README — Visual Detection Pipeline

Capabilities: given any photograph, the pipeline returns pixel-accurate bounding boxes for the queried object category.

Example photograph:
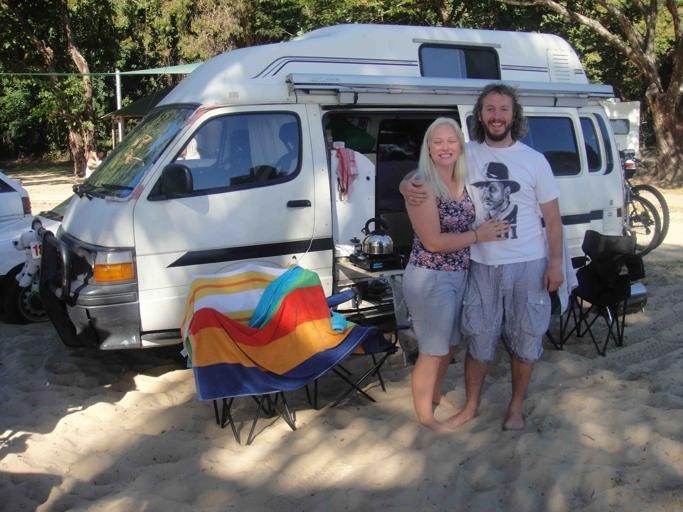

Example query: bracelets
[471,228,478,244]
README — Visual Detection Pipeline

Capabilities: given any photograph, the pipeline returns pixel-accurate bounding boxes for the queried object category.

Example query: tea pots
[361,218,394,255]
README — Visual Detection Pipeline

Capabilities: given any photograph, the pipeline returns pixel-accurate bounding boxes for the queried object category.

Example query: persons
[396,79,567,435]
[84,147,107,179]
[469,162,520,241]
[399,115,510,436]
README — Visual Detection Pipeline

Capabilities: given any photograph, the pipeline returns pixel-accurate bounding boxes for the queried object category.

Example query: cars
[0,192,75,326]
[1,170,35,228]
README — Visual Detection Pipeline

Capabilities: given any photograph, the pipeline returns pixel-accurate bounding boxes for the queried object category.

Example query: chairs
[185,263,394,447]
[274,122,299,174]
[562,230,646,358]
[230,128,251,172]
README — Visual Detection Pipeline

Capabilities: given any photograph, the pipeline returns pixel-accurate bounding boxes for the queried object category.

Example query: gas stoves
[350,249,402,271]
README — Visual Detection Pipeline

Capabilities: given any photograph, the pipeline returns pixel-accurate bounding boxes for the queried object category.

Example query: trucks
[43,28,625,372]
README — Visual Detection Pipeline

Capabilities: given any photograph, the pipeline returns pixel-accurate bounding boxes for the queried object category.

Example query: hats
[471,162,520,194]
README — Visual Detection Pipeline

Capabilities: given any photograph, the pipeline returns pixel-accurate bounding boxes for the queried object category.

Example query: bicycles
[616,146,673,260]
